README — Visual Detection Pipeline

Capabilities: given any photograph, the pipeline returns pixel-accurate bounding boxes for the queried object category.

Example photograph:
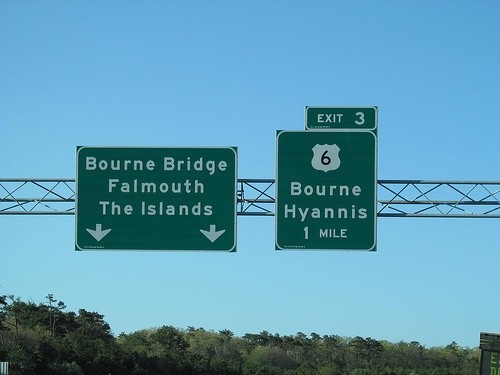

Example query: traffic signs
[275,106,379,251]
[75,144,239,252]
